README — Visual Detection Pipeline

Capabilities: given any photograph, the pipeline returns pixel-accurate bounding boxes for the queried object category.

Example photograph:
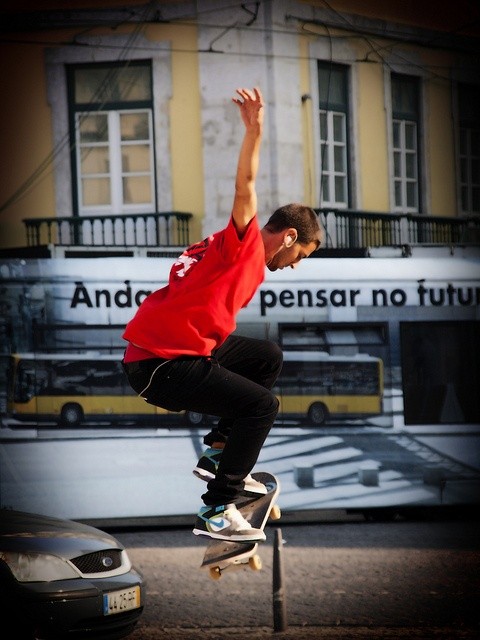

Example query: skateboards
[200,471,281,580]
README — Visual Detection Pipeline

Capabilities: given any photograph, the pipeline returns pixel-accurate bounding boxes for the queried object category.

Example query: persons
[122,84,322,543]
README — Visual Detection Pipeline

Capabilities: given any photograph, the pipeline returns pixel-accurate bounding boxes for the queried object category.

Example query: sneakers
[192,449,268,497]
[193,501,267,543]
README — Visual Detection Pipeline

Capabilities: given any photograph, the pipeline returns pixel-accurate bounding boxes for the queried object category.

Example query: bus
[9,349,383,430]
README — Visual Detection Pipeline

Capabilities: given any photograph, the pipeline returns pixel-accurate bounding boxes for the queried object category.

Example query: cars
[0,505,143,640]
